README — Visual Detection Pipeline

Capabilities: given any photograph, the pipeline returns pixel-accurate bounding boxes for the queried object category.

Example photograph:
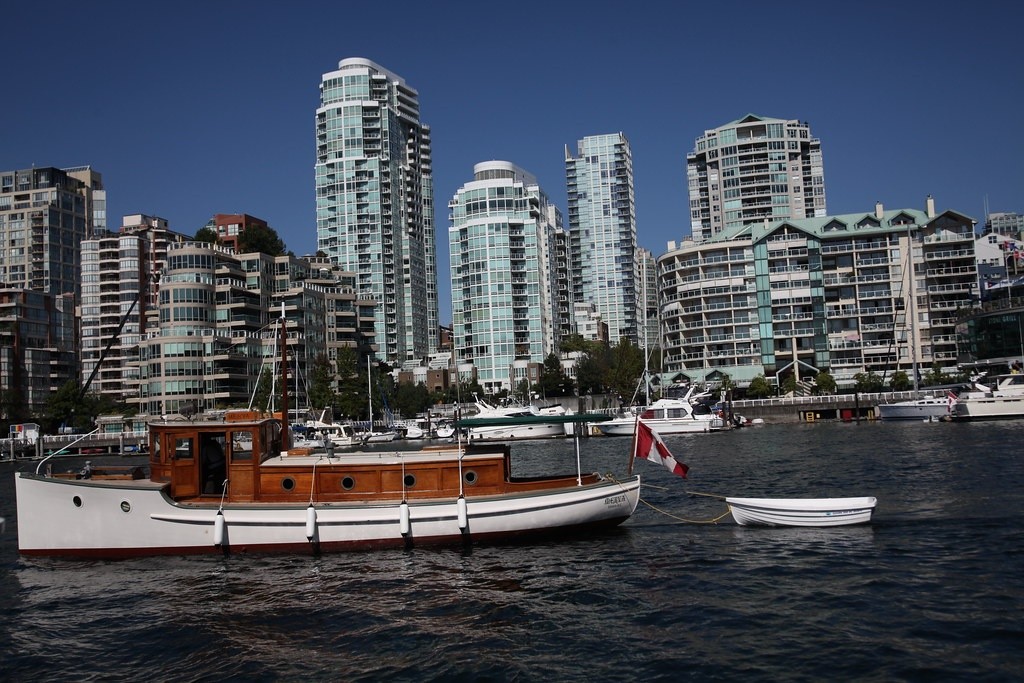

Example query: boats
[15,232,642,562]
[724,496,878,527]
[42,291,754,457]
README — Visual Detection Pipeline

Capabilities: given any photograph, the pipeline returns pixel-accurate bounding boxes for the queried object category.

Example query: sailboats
[873,223,1024,423]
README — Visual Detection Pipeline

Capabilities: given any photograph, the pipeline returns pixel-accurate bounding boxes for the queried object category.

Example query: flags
[1004,240,1015,252]
[1013,246,1024,261]
[635,415,690,482]
[946,390,962,413]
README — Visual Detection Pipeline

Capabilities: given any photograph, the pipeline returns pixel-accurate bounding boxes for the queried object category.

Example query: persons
[81,461,94,479]
[235,443,243,451]
